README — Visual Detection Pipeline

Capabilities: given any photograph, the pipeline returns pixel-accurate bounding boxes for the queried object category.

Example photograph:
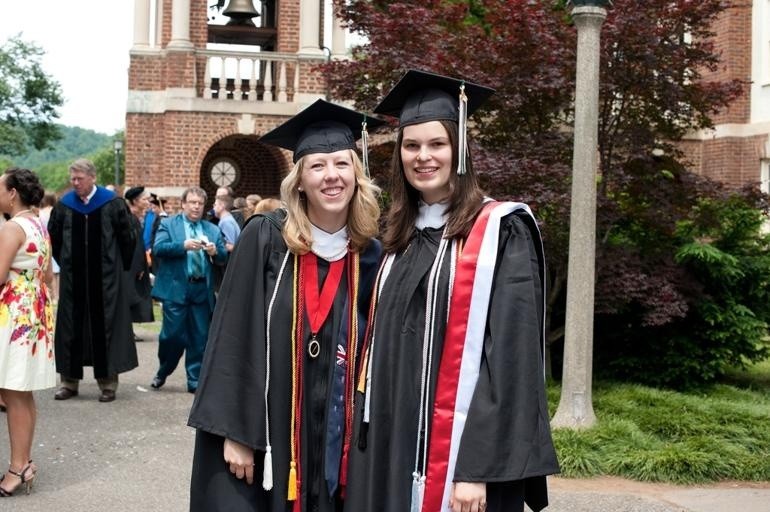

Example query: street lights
[113,139,124,185]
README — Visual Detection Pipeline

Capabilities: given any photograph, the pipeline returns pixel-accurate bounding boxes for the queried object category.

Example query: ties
[190,224,202,280]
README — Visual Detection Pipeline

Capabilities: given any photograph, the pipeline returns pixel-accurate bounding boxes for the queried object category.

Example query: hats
[125,186,144,200]
[256,98,386,182]
[372,69,497,177]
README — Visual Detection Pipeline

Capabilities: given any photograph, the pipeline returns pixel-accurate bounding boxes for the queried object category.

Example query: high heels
[0,459,37,497]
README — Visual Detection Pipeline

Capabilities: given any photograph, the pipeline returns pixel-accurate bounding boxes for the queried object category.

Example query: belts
[189,277,206,283]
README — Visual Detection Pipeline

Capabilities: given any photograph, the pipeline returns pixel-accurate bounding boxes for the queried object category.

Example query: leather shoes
[151,376,165,388]
[55,387,78,400]
[100,389,115,402]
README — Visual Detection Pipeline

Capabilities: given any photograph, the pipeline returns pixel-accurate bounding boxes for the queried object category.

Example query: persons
[1,164,155,411]
[151,187,285,391]
[342,121,560,512]
[1,165,58,499]
[191,148,388,512]
